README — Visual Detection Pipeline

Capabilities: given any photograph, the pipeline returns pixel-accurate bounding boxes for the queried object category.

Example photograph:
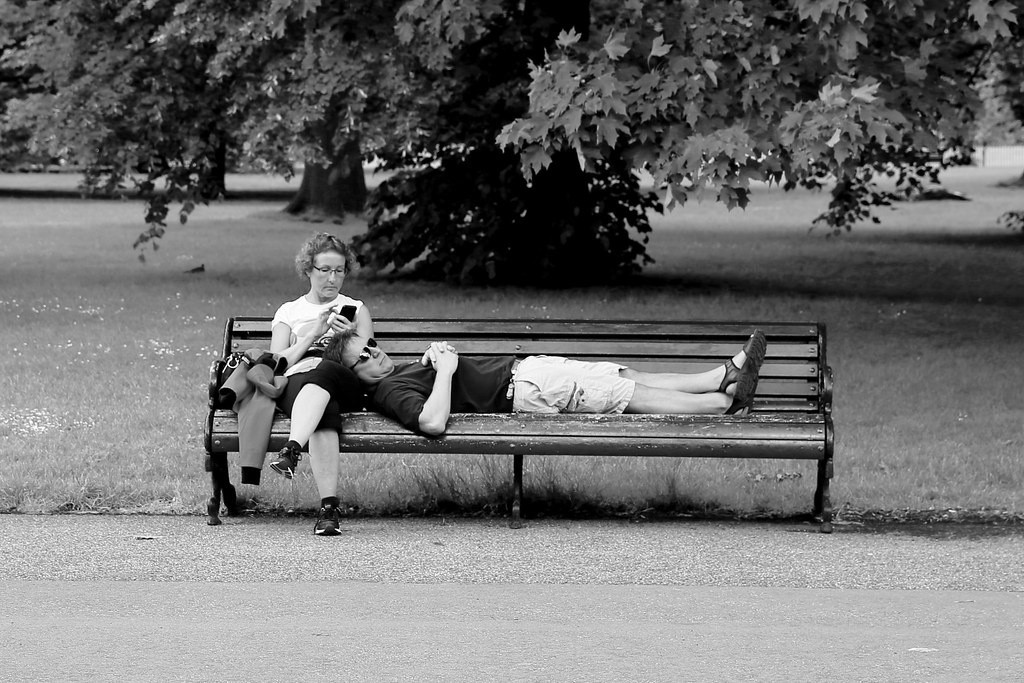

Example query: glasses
[313,266,349,276]
[349,338,377,370]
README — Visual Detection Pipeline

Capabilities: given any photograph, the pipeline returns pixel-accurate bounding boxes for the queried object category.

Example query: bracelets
[425,346,431,351]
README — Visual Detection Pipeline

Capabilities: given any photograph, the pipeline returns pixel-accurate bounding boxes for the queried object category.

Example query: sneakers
[314,505,342,536]
[269,445,301,479]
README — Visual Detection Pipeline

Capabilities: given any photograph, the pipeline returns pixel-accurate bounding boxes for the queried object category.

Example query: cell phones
[336,305,357,324]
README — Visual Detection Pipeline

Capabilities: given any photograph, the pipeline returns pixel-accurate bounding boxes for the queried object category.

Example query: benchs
[203,315,836,535]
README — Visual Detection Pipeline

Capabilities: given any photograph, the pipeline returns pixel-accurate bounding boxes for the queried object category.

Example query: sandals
[719,328,767,415]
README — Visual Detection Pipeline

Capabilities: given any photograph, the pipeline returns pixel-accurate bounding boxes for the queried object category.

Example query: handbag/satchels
[218,352,253,408]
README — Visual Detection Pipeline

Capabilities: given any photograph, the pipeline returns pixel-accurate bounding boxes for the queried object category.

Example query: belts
[510,358,522,380]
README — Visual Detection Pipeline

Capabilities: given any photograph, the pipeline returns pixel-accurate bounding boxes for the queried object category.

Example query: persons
[323,327,768,438]
[269,234,375,535]
